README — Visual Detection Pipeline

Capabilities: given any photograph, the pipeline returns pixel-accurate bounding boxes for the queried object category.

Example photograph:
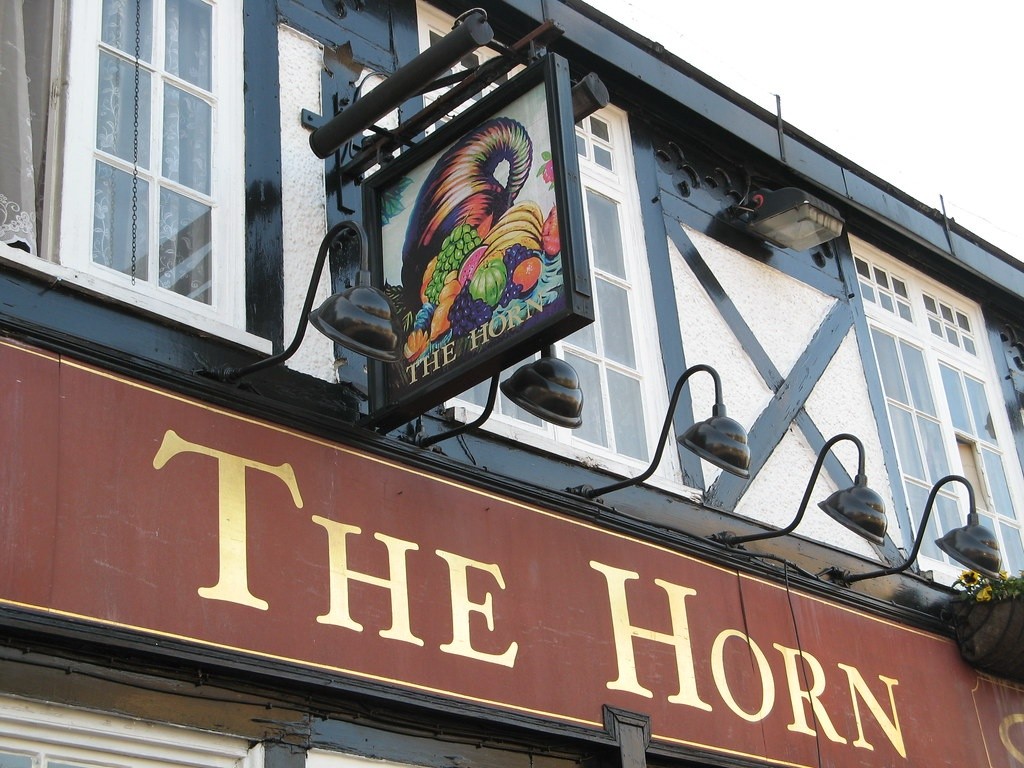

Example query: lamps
[727,184,845,250]
[416,337,582,449]
[710,434,887,552]
[564,364,752,497]
[817,475,1002,581]
[192,222,399,385]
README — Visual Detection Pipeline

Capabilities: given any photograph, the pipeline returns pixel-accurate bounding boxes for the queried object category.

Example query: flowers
[955,569,1024,599]
